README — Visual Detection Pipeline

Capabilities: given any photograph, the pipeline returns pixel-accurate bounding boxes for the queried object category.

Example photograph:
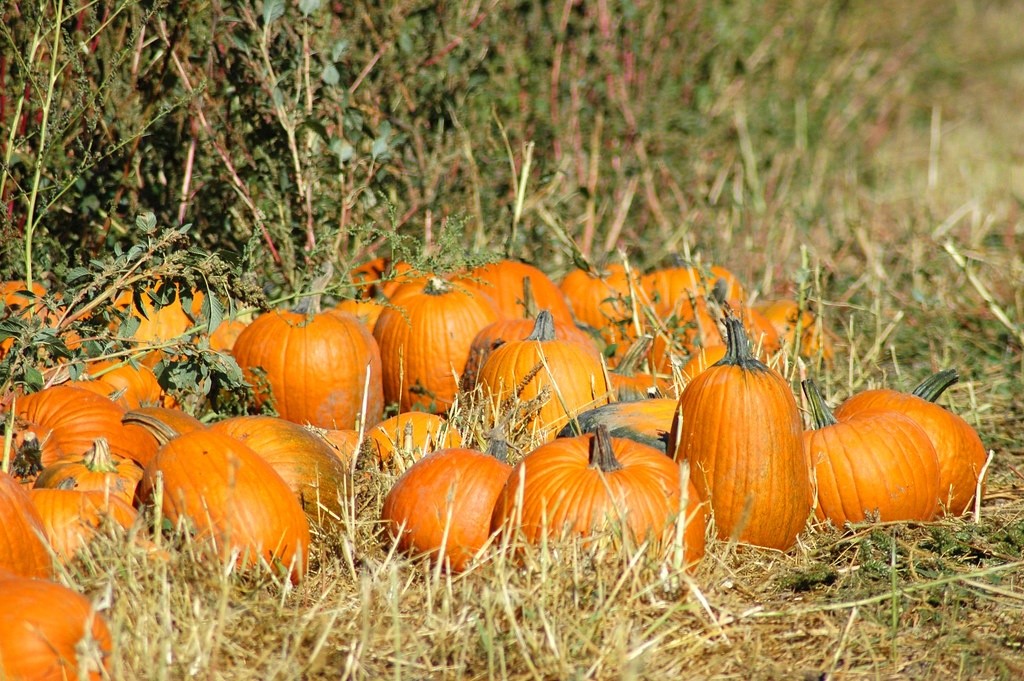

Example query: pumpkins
[0,261,835,681]
[797,378,939,532]
[830,368,988,519]
